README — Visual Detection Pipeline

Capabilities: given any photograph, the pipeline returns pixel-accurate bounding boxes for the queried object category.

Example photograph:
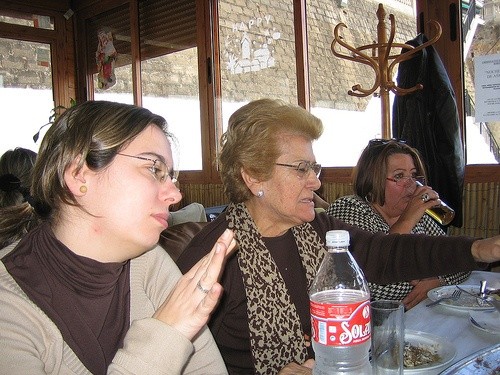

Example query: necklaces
[271,236,290,270]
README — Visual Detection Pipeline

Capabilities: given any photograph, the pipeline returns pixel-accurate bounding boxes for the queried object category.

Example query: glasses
[368,138,407,150]
[275,162,323,180]
[114,151,183,194]
[387,176,426,187]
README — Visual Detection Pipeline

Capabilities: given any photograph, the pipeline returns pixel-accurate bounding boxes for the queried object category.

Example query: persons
[0,101,236,375]
[326,138,471,310]
[175,99,500,375]
[0,147,39,249]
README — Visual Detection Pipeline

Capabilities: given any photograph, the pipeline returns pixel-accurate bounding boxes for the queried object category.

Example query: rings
[421,194,429,202]
[197,280,210,294]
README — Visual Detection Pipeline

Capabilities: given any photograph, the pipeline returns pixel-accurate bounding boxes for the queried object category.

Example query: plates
[469,309,500,335]
[436,343,500,375]
[427,285,500,311]
[376,328,457,372]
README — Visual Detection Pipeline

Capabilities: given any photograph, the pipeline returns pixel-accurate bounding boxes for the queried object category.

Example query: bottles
[307,229,374,375]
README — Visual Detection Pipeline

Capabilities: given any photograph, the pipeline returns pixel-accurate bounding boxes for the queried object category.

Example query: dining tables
[404,270,500,375]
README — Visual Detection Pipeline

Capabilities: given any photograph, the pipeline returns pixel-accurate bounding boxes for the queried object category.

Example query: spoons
[456,285,498,307]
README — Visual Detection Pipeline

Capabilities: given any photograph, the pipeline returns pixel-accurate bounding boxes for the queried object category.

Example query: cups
[407,181,455,226]
[371,300,405,375]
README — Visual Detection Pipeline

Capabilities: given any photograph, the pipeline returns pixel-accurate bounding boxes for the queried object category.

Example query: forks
[425,289,462,307]
